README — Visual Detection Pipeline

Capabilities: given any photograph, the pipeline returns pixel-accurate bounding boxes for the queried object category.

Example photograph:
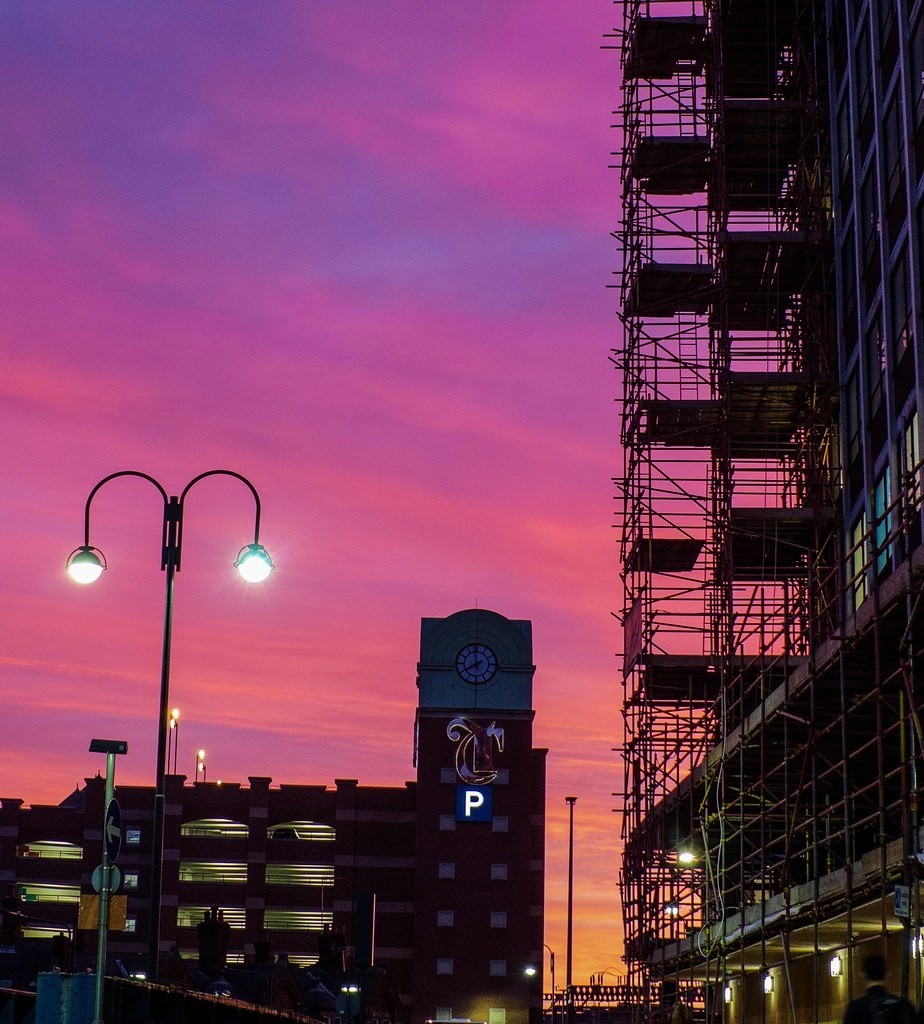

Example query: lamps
[723,987,734,1006]
[763,975,775,994]
[678,851,697,867]
[912,935,924,959]
[829,957,843,977]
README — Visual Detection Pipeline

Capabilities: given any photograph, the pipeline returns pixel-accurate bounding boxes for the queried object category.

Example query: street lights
[63,469,276,981]
[525,943,555,1024]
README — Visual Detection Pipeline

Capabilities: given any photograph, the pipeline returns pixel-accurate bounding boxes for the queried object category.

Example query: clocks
[452,640,500,685]
